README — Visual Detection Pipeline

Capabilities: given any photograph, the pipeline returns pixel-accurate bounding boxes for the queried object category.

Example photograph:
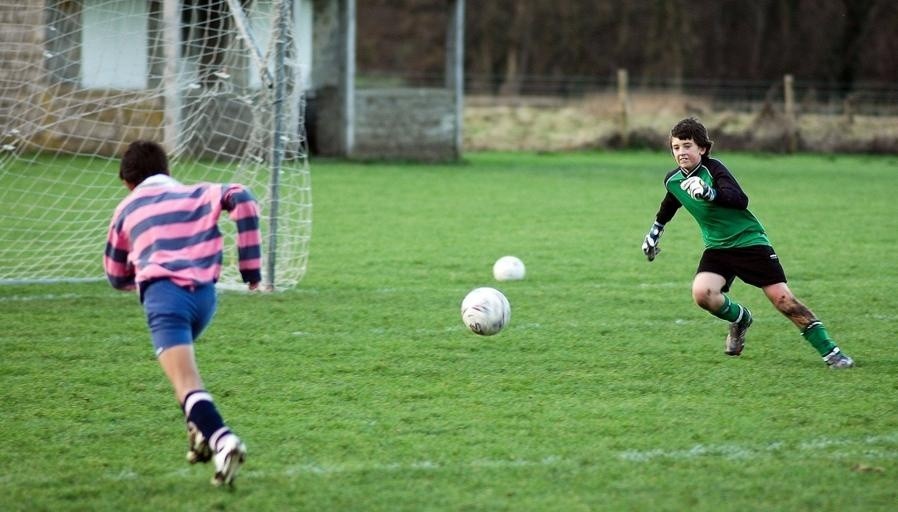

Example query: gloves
[680,176,715,202]
[641,221,665,263]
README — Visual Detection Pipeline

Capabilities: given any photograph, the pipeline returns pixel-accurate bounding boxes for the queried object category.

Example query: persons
[102,139,261,486]
[641,117,854,369]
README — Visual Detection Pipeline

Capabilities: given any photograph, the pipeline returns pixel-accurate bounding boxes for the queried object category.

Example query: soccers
[461,287,511,336]
[492,256,525,281]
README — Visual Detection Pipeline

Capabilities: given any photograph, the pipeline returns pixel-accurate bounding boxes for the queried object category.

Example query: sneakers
[184,421,213,464]
[210,433,248,486]
[826,355,855,368]
[725,304,754,356]
